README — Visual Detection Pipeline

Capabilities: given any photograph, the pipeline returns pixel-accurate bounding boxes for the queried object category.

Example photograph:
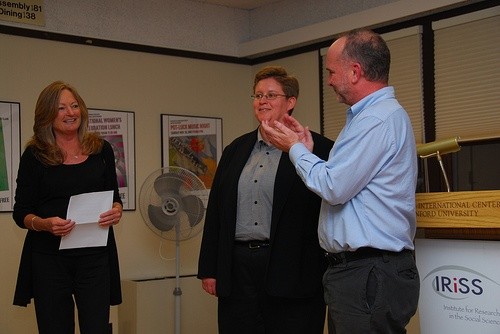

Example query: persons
[13,80,123,334]
[197,65,335,334]
[260,30,420,334]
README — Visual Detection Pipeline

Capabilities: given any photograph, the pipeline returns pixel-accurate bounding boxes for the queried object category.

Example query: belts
[323,248,412,266]
[233,240,272,248]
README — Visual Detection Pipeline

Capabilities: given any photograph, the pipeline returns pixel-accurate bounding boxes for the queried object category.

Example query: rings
[62,233,64,236]
[112,220,115,223]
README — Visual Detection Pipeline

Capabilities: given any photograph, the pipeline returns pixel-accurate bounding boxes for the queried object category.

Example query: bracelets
[32,216,40,232]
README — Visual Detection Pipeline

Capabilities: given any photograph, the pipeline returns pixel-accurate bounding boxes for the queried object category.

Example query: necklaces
[59,143,81,159]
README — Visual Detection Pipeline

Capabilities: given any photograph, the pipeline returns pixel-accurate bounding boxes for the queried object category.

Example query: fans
[138,166,209,334]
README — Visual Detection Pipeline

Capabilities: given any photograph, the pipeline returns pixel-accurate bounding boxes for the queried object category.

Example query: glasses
[251,92,291,100]
